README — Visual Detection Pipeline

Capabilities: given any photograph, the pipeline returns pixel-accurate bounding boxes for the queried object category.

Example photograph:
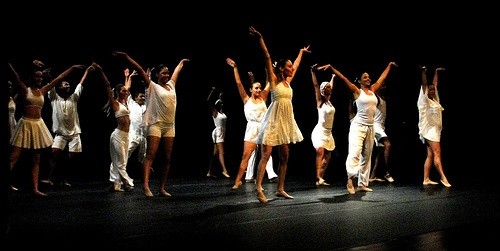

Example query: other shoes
[318,178,326,185]
[11,186,18,190]
[142,191,154,197]
[222,172,231,178]
[347,184,355,195]
[423,180,438,185]
[369,176,385,182]
[35,191,48,196]
[42,180,53,185]
[316,181,331,185]
[440,179,451,188]
[384,173,394,182]
[114,187,124,192]
[357,187,374,192]
[160,190,171,197]
[257,194,267,202]
[276,191,294,199]
[206,173,211,177]
[232,181,242,189]
[126,186,134,192]
[245,178,256,182]
[64,181,71,186]
[269,177,279,182]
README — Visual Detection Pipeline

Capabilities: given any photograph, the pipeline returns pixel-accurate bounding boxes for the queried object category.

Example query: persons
[311,63,336,186]
[418,66,452,189]
[316,62,398,195]
[206,87,232,178]
[111,51,190,198]
[225,57,280,192]
[249,25,311,203]
[3,59,154,197]
